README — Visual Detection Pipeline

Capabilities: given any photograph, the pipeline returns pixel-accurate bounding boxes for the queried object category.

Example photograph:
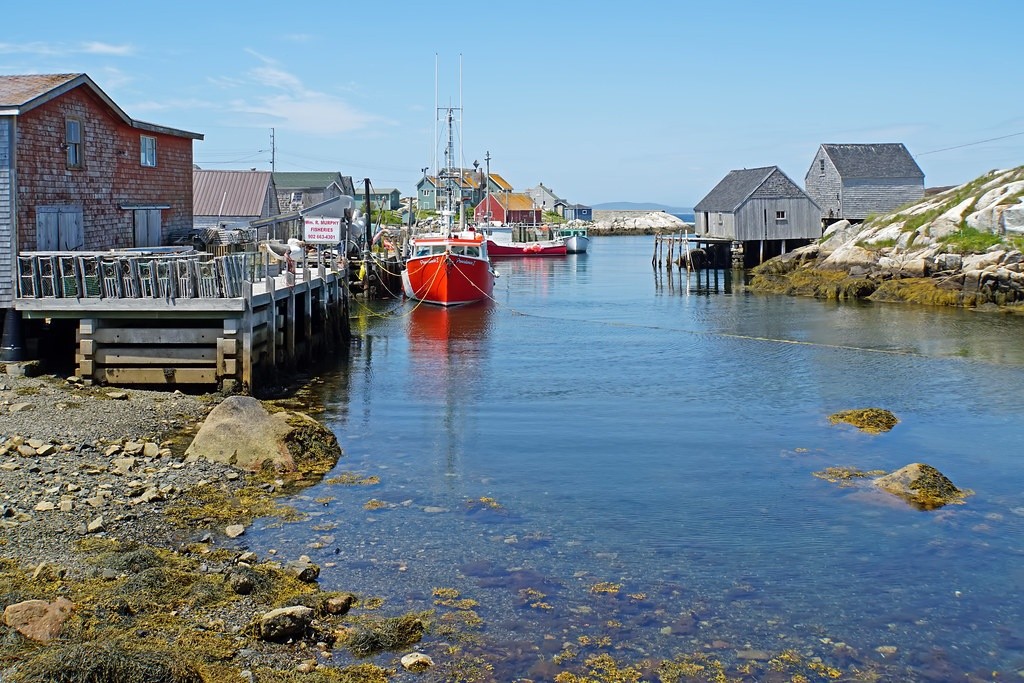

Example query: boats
[401,50,500,308]
[471,151,568,257]
[556,200,589,254]
